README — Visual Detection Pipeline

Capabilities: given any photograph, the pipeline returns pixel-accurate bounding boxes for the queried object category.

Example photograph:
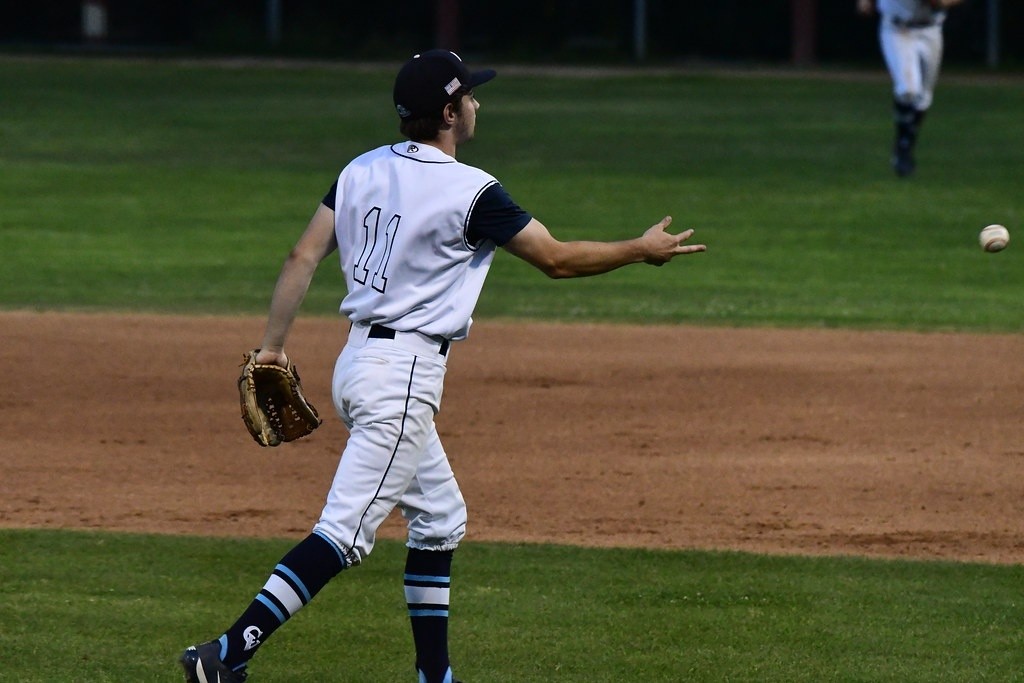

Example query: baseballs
[980,224,1010,252]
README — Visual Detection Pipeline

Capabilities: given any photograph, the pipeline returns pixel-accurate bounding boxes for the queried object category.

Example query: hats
[393,49,497,121]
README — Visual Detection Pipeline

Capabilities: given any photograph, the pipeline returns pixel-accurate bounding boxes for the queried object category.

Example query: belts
[892,16,935,29]
[349,321,449,356]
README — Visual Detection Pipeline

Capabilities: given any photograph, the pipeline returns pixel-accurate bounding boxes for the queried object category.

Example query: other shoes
[182,639,249,683]
[894,139,917,179]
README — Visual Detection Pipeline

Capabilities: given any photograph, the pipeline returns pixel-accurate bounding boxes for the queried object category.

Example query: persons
[858,0,960,179]
[181,50,706,683]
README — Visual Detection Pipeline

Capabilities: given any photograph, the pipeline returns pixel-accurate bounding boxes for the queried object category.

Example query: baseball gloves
[238,348,323,449]
